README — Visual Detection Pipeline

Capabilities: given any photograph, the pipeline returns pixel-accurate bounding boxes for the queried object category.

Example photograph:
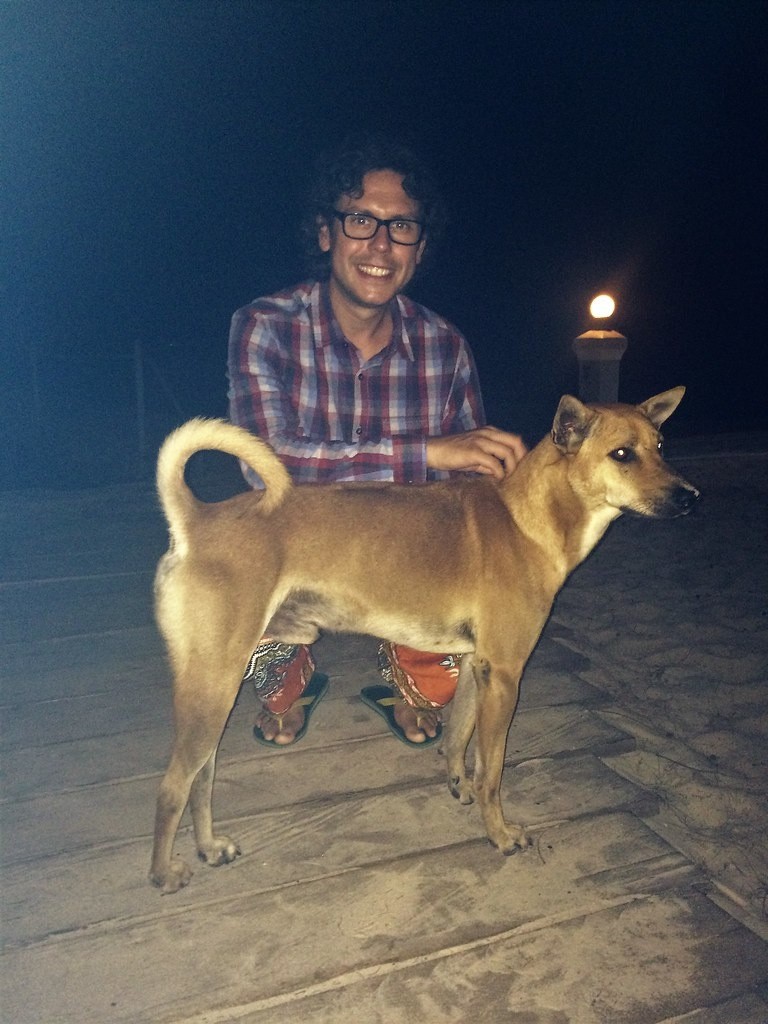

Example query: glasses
[328,205,425,246]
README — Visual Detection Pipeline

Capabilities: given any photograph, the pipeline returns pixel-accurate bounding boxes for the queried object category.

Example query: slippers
[360,684,443,749]
[252,670,330,748]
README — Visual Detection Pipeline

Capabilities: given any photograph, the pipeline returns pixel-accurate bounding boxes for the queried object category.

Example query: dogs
[148,385,700,895]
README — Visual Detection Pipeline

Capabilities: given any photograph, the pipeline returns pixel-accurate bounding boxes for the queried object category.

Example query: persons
[227,153,529,749]
